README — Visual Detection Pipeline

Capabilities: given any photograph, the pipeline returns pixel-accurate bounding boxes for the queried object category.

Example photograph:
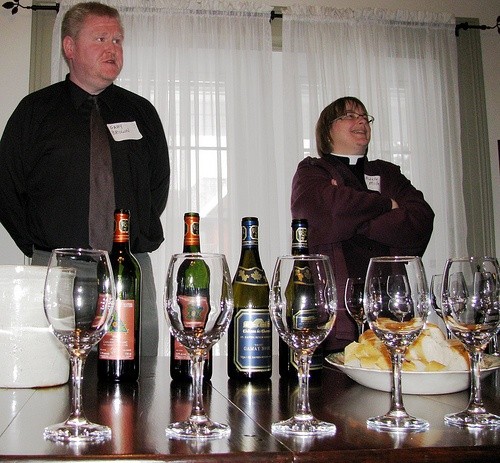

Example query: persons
[0,2,170,358]
[291,97,435,367]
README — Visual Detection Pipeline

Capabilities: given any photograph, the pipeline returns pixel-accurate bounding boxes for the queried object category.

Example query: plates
[326,349,499,395]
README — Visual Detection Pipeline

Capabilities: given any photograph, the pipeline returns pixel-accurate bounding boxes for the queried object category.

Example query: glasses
[331,111,374,123]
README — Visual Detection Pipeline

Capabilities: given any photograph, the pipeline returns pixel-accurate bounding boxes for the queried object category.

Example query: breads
[343,316,471,373]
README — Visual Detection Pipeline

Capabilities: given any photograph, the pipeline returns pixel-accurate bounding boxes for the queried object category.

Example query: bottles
[96,378,136,453]
[170,379,212,454]
[226,377,272,452]
[229,217,272,378]
[279,219,325,374]
[170,212,212,380]
[99,208,141,382]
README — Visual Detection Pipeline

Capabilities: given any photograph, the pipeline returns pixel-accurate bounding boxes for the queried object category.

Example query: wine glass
[44,248,117,447]
[430,272,467,338]
[269,255,338,436]
[164,253,233,442]
[440,258,499,430]
[345,278,382,341]
[364,257,430,432]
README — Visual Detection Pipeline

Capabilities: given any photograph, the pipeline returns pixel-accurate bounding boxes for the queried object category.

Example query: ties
[88,94,116,262]
